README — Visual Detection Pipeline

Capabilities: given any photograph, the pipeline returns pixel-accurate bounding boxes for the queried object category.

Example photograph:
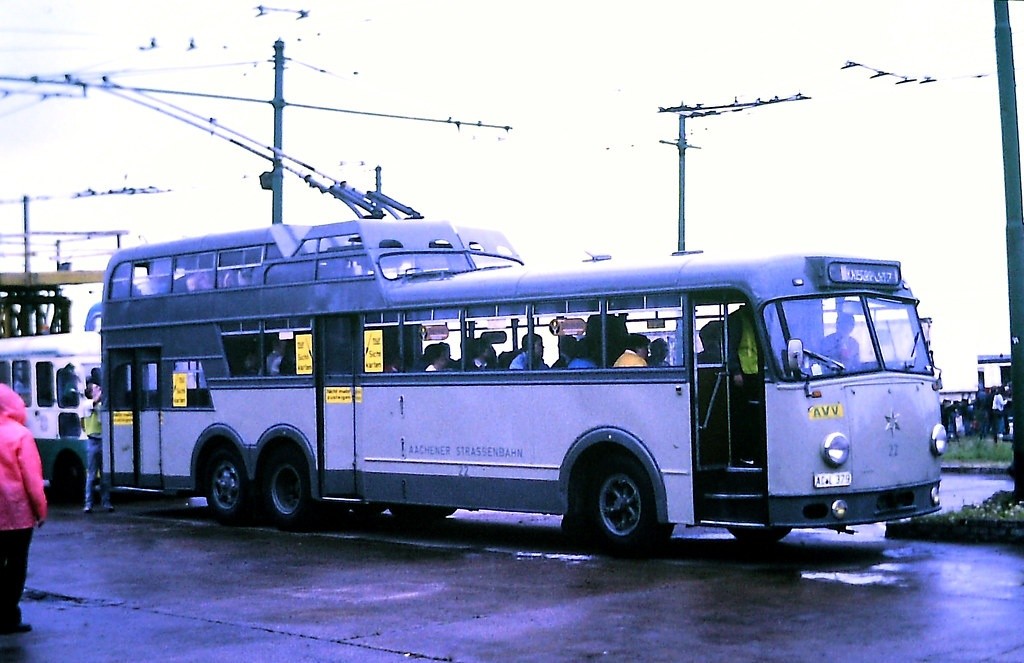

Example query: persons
[612,334,650,369]
[380,338,499,374]
[0,383,49,634]
[257,334,285,376]
[81,383,114,513]
[819,314,860,364]
[551,335,577,368]
[726,300,762,468]
[646,338,671,367]
[568,337,597,367]
[586,313,629,336]
[509,333,550,370]
[940,382,1013,442]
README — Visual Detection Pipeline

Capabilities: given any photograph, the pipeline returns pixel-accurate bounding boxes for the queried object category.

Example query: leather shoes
[0,618,32,638]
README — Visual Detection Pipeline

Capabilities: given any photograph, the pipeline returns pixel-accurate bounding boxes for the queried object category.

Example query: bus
[2,331,103,501]
[96,216,947,553]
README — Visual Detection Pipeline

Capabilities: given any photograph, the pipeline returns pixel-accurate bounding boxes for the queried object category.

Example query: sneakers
[84,505,91,513]
[103,502,113,509]
